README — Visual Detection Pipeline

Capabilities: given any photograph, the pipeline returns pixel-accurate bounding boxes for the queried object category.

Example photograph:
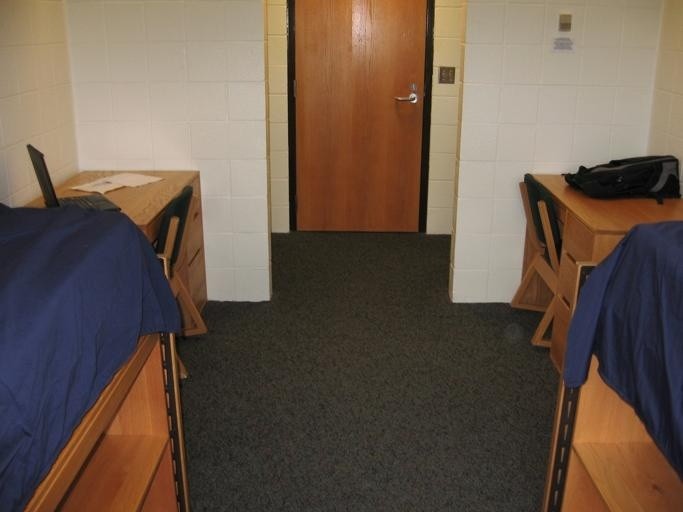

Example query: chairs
[508,172,561,348]
[153,185,209,381]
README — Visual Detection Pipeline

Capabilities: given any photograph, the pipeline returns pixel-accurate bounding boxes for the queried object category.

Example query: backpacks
[564,156,681,205]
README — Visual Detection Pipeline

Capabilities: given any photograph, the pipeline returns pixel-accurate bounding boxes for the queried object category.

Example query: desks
[23,169,208,317]
[518,172,683,376]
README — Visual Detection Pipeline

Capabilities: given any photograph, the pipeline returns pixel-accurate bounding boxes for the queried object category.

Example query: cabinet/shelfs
[22,332,191,512]
[541,259,682,512]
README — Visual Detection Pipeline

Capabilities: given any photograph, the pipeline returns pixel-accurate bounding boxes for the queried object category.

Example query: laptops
[27,144,122,212]
